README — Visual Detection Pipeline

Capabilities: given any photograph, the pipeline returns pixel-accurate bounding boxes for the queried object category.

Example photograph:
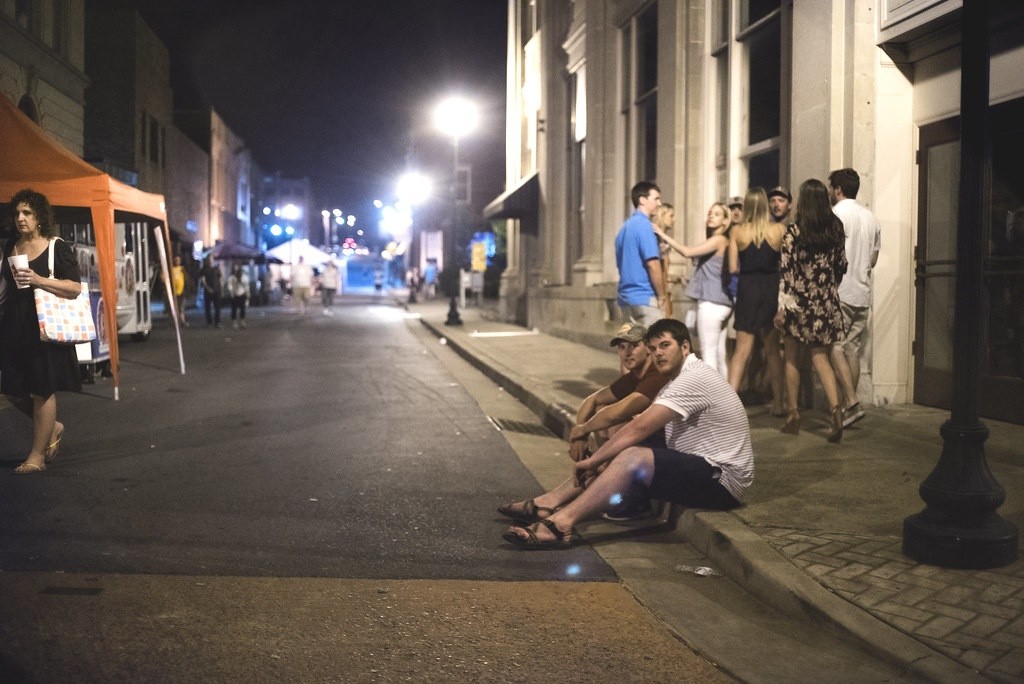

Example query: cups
[7,254,31,288]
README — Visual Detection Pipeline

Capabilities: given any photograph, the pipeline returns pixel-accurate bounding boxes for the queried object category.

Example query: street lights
[401,172,430,303]
[436,97,483,329]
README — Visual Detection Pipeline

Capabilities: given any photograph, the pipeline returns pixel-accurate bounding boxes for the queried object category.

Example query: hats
[769,186,791,202]
[610,323,648,347]
[727,196,743,207]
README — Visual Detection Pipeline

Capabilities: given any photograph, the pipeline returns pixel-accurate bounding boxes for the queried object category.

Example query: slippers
[46,429,64,461]
[12,463,46,476]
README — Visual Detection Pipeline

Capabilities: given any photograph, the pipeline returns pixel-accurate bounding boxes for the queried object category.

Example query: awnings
[482,173,539,220]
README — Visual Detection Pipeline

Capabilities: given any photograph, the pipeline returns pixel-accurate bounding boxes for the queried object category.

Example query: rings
[28,276,29,280]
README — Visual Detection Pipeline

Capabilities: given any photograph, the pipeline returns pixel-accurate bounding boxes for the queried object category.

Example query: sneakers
[603,491,652,520]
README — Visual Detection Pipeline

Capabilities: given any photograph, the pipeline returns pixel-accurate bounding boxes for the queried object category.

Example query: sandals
[503,519,573,549]
[497,499,553,524]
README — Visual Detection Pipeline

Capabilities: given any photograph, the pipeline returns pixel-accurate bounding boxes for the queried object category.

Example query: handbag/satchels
[35,237,97,345]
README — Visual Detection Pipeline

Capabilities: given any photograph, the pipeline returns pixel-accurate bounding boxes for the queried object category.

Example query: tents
[0,88,185,401]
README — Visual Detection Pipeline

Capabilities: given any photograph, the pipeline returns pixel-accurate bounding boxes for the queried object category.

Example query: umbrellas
[202,239,284,264]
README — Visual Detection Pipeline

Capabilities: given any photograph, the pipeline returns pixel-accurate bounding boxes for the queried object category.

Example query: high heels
[827,405,844,441]
[781,408,801,435]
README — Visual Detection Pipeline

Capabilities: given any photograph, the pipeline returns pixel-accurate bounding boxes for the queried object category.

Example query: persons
[0,190,82,474]
[172,256,192,328]
[828,167,880,429]
[613,181,677,374]
[406,260,437,303]
[374,265,383,300]
[227,262,251,328]
[652,202,735,381]
[499,318,754,548]
[773,179,848,443]
[201,253,225,328]
[291,255,314,313]
[321,260,337,319]
[570,323,669,522]
[727,186,794,414]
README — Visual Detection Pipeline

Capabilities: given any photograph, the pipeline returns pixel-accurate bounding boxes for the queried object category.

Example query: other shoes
[233,321,239,329]
[240,318,247,328]
[214,321,222,328]
[323,308,332,316]
[842,402,866,427]
